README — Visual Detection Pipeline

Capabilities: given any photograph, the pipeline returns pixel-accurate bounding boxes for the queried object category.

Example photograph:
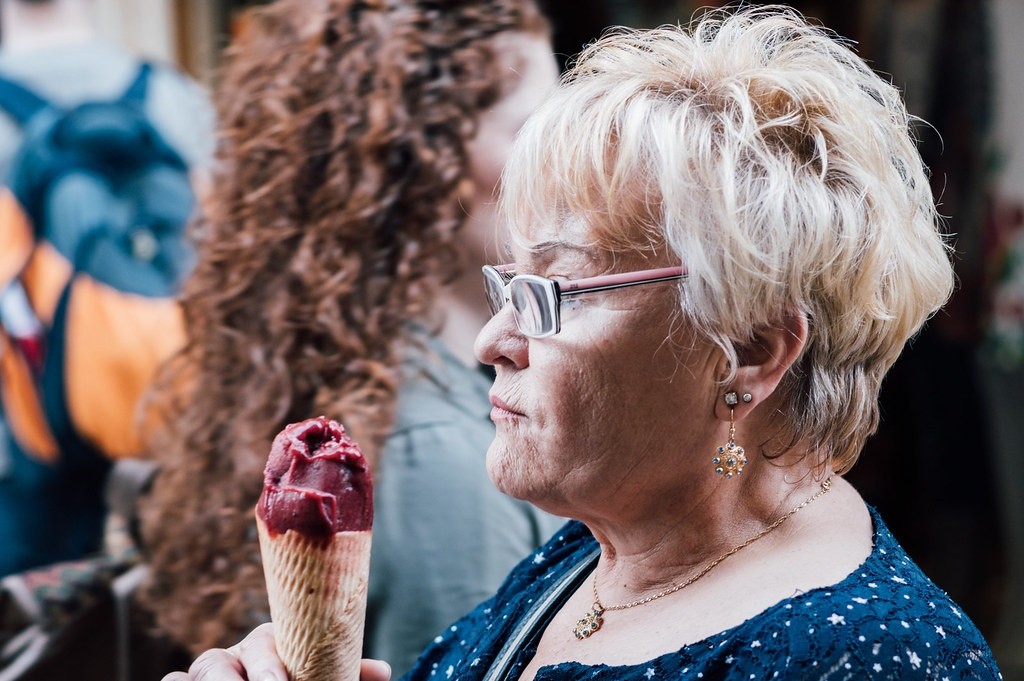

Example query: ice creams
[255,414,373,681]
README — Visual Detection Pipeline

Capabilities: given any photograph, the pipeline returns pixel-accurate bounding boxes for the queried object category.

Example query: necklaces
[572,475,831,641]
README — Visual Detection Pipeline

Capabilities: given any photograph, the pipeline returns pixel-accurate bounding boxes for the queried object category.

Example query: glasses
[482,263,744,338]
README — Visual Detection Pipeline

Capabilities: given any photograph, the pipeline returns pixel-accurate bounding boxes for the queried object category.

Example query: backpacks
[0,59,210,460]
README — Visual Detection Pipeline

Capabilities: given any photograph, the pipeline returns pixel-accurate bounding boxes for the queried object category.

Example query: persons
[160,5,1003,681]
[134,0,563,681]
[0,0,216,576]
